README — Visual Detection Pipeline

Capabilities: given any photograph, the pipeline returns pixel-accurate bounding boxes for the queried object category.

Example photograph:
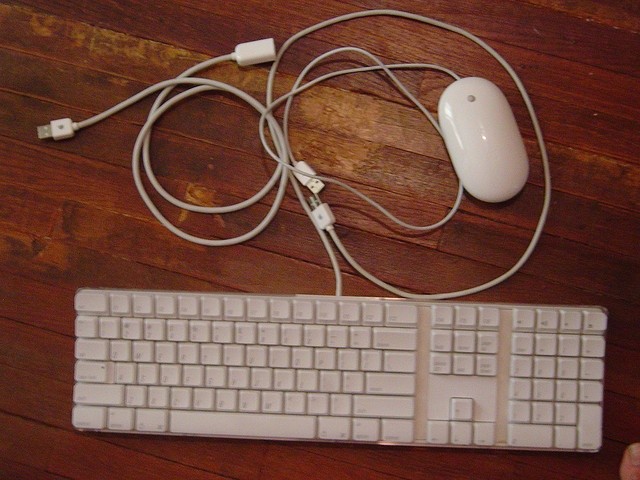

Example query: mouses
[436,76,530,204]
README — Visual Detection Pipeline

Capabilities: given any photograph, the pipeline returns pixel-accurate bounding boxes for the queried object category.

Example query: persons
[619,442,640,480]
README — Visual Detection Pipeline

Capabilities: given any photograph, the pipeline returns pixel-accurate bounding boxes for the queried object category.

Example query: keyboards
[70,283,611,459]
